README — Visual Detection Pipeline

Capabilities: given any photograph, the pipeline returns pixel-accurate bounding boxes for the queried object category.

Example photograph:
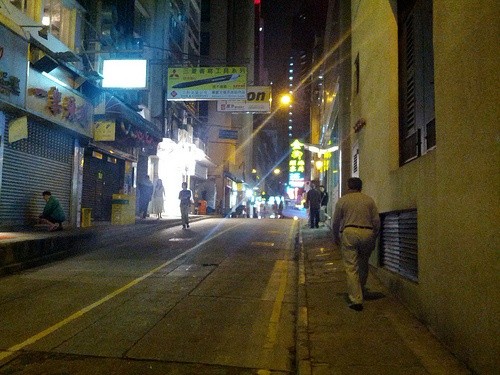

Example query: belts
[344,225,373,230]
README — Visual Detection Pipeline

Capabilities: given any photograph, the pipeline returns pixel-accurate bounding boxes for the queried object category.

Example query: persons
[318,185,331,227]
[153,179,165,219]
[273,201,278,219]
[278,200,284,218]
[332,177,381,311]
[179,182,191,229]
[139,175,153,219]
[38,191,65,230]
[306,183,321,229]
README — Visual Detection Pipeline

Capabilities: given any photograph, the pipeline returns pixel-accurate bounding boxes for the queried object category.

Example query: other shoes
[48,223,59,232]
[187,225,189,227]
[348,303,362,311]
[183,226,186,229]
